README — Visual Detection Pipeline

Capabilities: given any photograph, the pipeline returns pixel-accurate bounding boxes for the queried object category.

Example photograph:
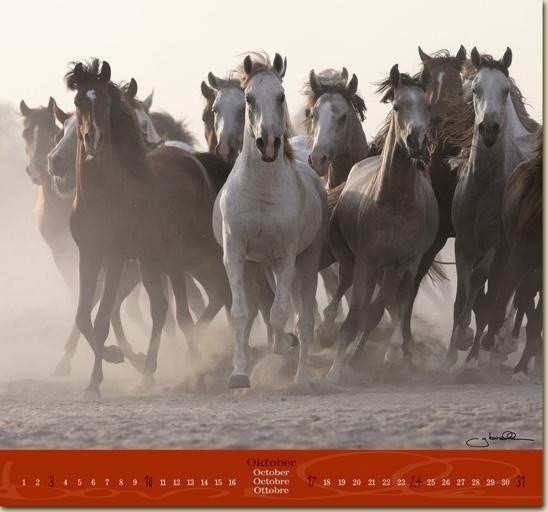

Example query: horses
[11,45,544,406]
